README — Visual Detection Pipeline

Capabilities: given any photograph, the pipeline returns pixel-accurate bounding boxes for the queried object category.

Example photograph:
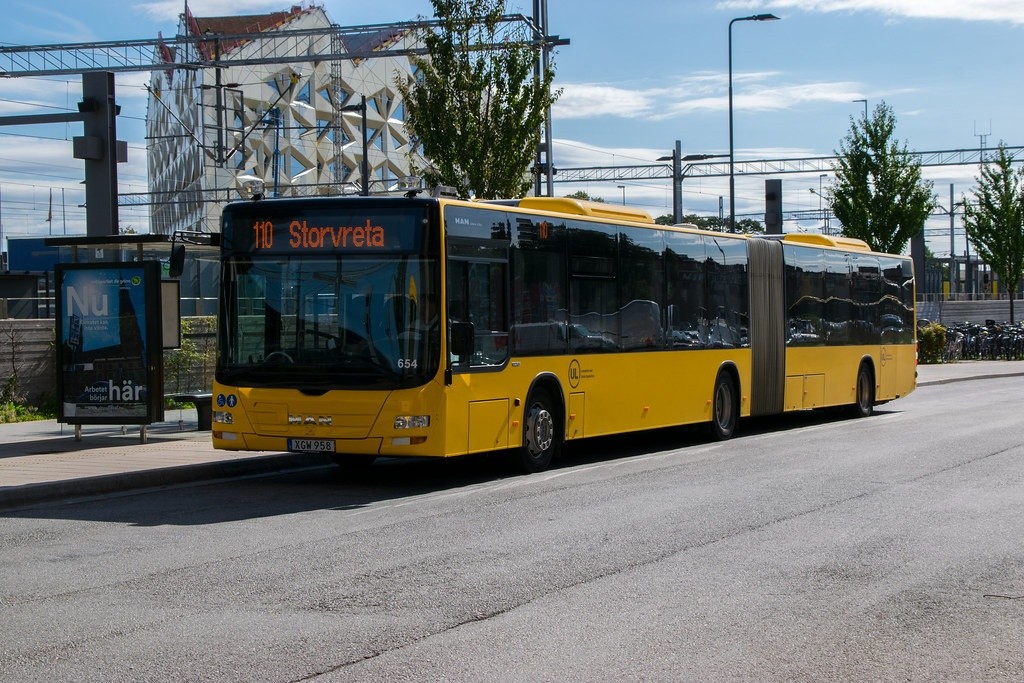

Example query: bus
[168,191,924,474]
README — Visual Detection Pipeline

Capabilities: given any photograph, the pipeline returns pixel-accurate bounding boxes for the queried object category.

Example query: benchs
[173,390,213,430]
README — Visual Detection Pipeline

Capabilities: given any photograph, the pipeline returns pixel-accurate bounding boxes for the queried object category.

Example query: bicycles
[917,317,1024,361]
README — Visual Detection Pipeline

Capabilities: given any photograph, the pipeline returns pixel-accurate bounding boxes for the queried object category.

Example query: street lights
[617,185,627,206]
[925,184,966,299]
[853,98,870,146]
[819,174,829,222]
[336,92,369,198]
[682,13,784,232]
